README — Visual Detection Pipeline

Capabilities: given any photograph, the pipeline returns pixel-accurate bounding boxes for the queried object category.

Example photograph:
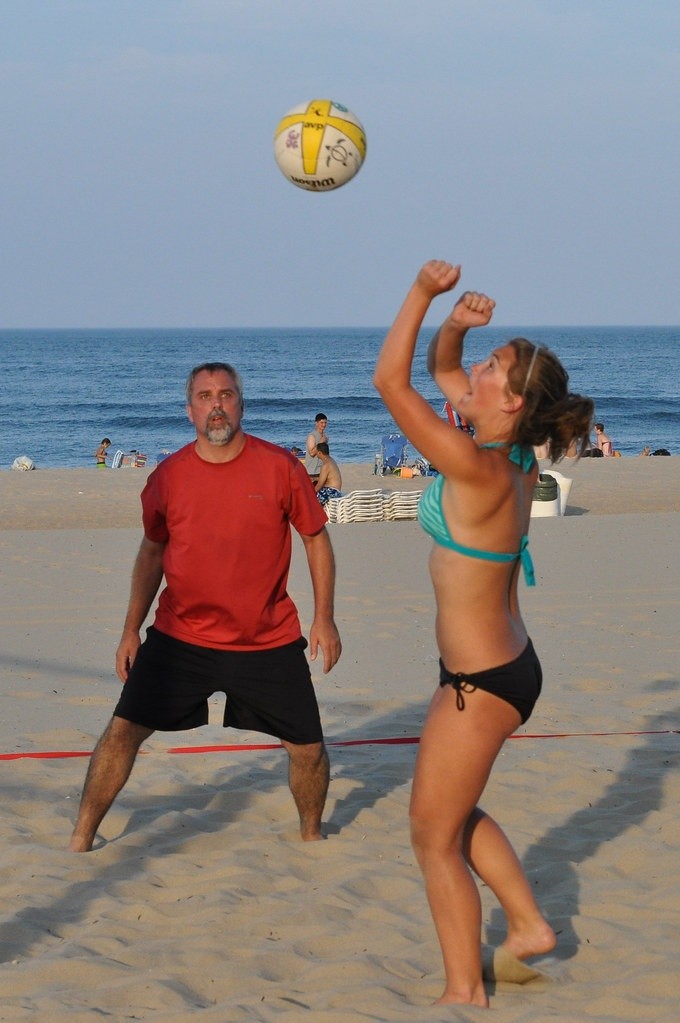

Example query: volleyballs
[272,97,369,194]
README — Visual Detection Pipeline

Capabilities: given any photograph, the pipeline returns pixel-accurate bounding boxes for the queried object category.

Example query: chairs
[376,435,408,478]
[322,489,423,525]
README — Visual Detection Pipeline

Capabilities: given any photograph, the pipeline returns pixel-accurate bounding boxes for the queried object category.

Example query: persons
[591,423,612,457]
[641,445,670,456]
[563,439,577,458]
[287,447,297,456]
[71,362,342,852]
[373,260,593,1005]
[580,441,604,458]
[305,413,329,487]
[96,438,111,468]
[314,443,342,505]
[156,449,171,464]
[534,443,550,459]
